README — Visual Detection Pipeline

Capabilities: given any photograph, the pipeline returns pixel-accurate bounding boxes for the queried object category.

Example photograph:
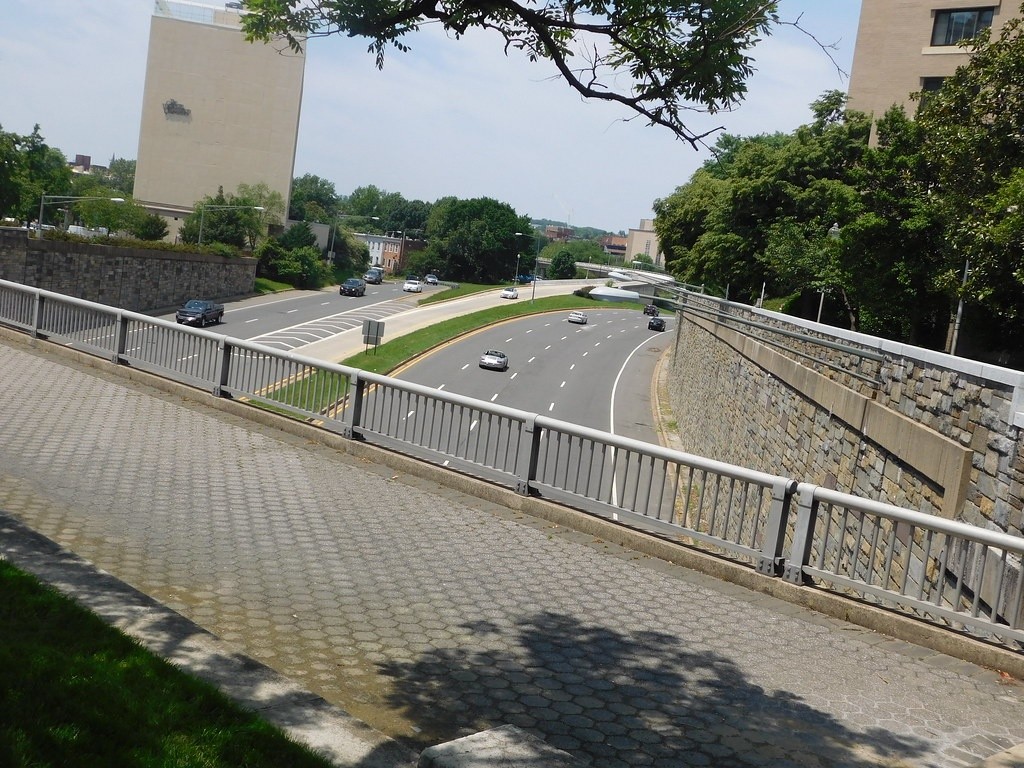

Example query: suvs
[424,275,438,285]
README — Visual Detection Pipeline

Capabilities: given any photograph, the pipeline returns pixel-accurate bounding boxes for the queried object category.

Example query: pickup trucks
[175,300,225,325]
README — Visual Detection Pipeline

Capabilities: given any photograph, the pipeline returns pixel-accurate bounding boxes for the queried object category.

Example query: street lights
[585,256,592,280]
[328,213,380,268]
[36,195,125,238]
[198,202,265,246]
[515,232,542,301]
[514,254,520,287]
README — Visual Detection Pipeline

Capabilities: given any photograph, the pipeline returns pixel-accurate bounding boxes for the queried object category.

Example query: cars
[643,304,661,317]
[478,349,508,373]
[500,288,519,299]
[340,278,366,297]
[403,280,423,293]
[568,311,588,324]
[648,318,666,332]
[363,269,380,284]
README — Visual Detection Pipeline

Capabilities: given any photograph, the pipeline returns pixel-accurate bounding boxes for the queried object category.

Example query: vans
[372,266,385,282]
